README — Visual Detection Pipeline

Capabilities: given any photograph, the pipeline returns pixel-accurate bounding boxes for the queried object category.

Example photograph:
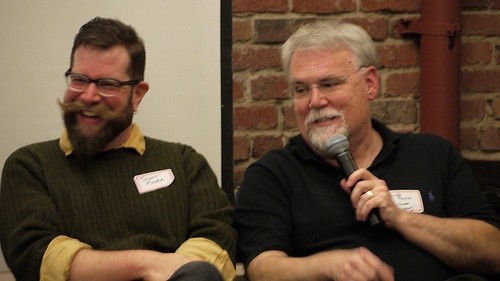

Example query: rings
[365,190,373,199]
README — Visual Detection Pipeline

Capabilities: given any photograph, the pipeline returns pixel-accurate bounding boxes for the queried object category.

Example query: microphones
[323,134,384,228]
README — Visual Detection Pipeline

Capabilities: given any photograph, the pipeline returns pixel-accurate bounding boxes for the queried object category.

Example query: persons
[0,16,236,281]
[234,20,500,281]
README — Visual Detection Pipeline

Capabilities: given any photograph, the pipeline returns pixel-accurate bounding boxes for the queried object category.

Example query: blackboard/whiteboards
[0,0,234,207]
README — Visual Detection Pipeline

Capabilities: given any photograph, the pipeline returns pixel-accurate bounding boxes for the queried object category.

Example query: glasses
[65,68,142,95]
[283,64,369,100]
